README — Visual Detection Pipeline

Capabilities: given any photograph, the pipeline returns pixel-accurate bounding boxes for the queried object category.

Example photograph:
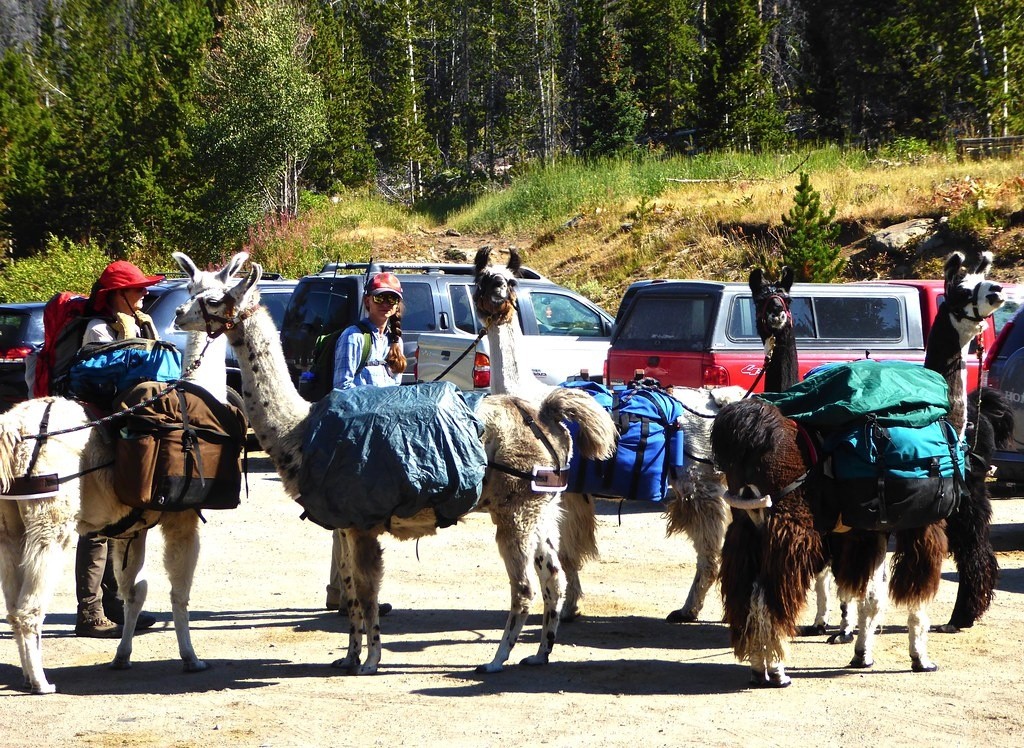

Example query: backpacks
[303,322,372,402]
[25,291,88,404]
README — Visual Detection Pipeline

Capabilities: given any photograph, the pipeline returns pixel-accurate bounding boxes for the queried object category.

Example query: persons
[74,259,157,638]
[325,273,409,616]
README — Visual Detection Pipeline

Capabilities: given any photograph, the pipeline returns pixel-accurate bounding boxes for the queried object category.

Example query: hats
[99,260,166,293]
[366,272,404,300]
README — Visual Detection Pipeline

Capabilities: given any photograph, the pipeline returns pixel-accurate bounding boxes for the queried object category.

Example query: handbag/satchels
[558,381,682,503]
[69,337,182,398]
[112,380,246,510]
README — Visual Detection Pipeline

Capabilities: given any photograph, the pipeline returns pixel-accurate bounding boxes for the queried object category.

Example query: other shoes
[104,605,156,630]
[338,603,391,618]
[76,618,132,638]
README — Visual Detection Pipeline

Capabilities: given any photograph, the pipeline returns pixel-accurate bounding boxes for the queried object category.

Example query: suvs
[281,261,615,403]
[971,305,1024,498]
[140,272,301,431]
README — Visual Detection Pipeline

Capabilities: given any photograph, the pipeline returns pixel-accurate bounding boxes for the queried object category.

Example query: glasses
[125,288,145,293]
[373,294,399,305]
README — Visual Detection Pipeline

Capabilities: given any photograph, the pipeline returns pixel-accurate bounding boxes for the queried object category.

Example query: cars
[0,302,50,414]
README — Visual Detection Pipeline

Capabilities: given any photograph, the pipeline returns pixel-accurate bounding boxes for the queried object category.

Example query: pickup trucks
[414,332,611,400]
[601,277,1023,483]
